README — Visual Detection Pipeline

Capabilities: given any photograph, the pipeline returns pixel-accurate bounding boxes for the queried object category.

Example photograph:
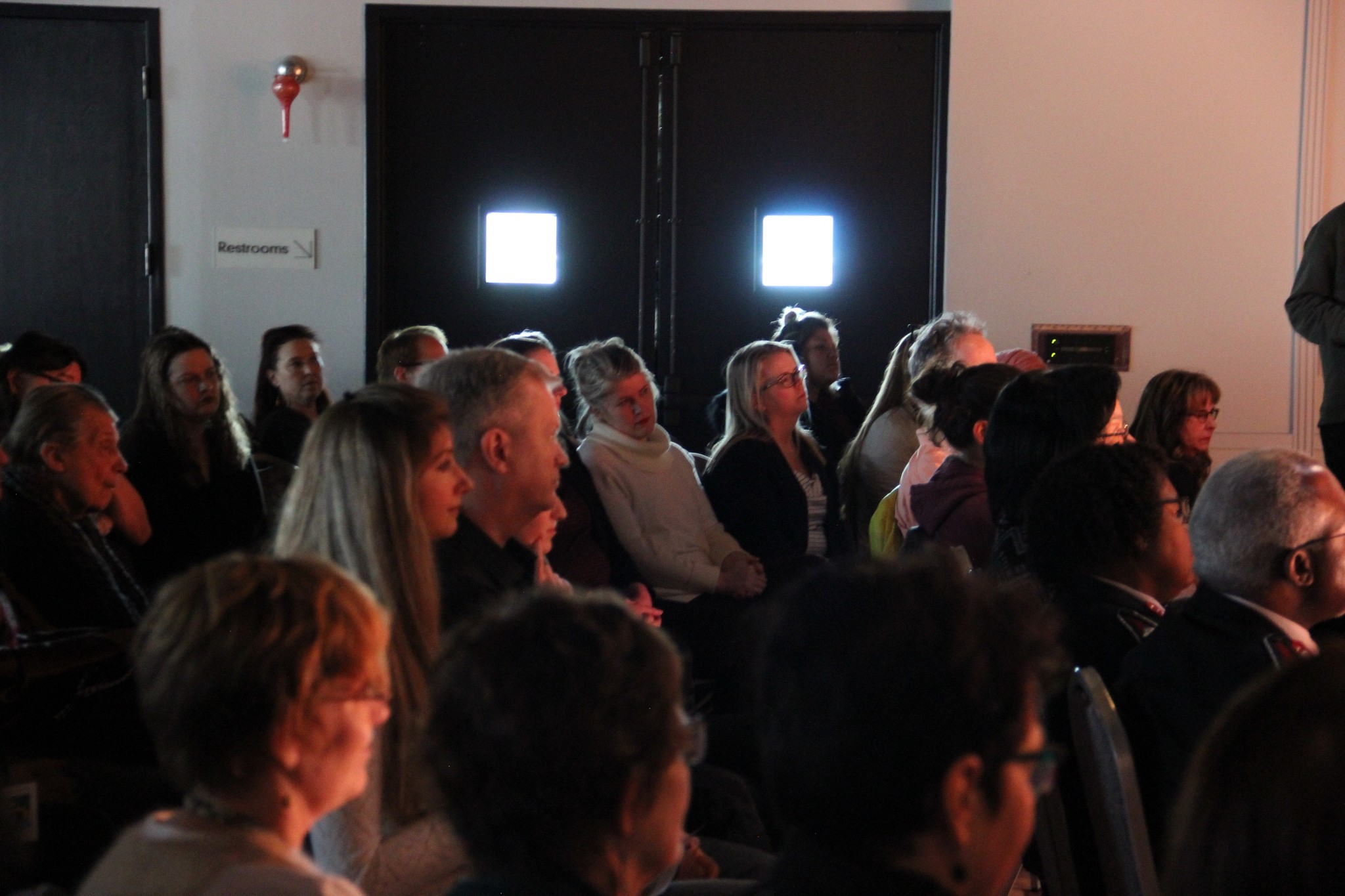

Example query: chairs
[1050,662,1171,896]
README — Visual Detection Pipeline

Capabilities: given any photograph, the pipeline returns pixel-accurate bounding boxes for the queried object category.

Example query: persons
[1285,203,1345,489]
[0,307,1345,896]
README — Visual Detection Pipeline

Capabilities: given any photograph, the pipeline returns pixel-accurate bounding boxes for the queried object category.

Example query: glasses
[1145,494,1190,521]
[758,363,809,395]
[1097,423,1129,440]
[1184,408,1219,424]
[649,719,707,767]
[997,747,1054,788]
[314,682,387,704]
